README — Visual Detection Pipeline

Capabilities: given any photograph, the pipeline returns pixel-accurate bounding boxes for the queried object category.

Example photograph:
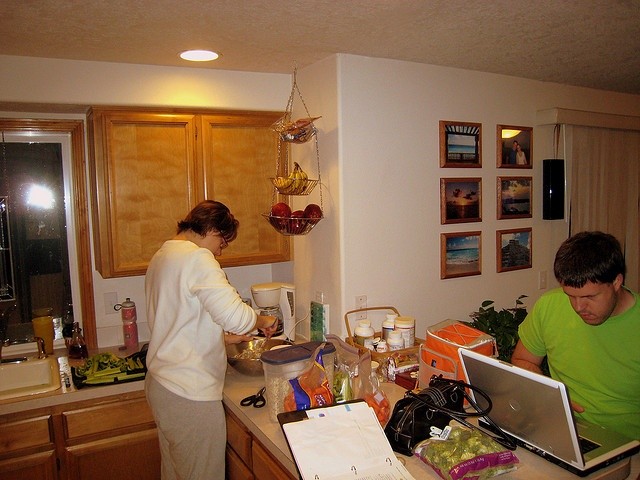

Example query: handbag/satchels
[383,373,517,457]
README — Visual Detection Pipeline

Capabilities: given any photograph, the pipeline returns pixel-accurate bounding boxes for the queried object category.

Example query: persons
[143,200,279,479]
[515,144,528,165]
[506,140,518,165]
[509,230,639,445]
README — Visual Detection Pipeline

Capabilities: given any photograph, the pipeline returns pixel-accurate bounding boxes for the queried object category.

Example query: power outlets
[103,291,119,314]
[354,295,367,320]
[539,270,548,289]
[314,289,323,303]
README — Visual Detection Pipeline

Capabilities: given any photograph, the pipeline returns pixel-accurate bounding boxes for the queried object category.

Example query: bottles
[68,321,90,369]
[394,316,414,349]
[62,303,73,339]
[372,337,381,351]
[387,330,404,352]
[376,338,388,354]
[354,318,375,347]
[57,356,75,393]
[52,317,64,340]
[383,312,399,341]
[121,298,139,350]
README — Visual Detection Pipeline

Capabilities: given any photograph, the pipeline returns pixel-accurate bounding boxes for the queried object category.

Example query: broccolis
[412,426,520,479]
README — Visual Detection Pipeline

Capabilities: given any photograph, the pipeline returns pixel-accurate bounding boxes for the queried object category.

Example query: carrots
[360,391,390,428]
[282,383,334,414]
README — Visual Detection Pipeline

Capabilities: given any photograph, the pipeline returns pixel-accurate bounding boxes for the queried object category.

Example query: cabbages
[330,370,355,404]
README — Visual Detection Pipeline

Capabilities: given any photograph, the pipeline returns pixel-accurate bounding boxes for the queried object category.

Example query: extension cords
[309,300,329,341]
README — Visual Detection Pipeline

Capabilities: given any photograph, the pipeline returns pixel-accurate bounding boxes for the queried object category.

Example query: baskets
[261,212,324,236]
[344,307,426,374]
[268,176,320,196]
[269,116,318,143]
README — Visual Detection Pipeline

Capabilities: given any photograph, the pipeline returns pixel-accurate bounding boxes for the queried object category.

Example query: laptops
[457,347,640,477]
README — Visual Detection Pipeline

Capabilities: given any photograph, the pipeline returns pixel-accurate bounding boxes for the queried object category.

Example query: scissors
[241,386,267,408]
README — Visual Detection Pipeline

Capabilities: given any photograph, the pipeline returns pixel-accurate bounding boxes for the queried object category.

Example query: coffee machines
[249,282,296,343]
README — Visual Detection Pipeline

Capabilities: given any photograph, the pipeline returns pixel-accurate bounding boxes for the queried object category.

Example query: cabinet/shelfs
[0,390,162,480]
[221,396,298,480]
[85,106,293,280]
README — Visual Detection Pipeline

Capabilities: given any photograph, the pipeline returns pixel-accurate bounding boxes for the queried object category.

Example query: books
[276,398,416,480]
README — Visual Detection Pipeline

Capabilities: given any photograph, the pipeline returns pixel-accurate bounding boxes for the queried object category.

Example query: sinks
[0,354,61,401]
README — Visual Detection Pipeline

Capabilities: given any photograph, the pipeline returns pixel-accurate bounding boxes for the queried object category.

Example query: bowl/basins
[224,338,296,377]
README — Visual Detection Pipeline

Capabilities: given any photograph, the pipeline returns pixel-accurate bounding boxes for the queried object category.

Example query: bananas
[271,161,309,195]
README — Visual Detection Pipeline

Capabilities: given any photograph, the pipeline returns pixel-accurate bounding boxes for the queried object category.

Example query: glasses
[219,229,228,249]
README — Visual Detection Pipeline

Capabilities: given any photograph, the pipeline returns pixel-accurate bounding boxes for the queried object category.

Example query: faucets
[32,335,47,360]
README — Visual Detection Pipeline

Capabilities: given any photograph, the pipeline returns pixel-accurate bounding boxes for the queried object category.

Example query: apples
[269,202,292,230]
[287,210,307,235]
[304,203,322,225]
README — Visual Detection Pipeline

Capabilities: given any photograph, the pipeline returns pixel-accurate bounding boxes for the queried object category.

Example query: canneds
[122,321,139,350]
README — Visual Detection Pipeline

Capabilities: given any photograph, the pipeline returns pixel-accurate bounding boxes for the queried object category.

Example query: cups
[32,316,54,356]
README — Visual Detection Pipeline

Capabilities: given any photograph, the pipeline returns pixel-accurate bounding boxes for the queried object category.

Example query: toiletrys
[69,322,88,360]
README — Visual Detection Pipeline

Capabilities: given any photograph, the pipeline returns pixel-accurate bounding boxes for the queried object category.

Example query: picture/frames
[439,119,483,169]
[440,230,483,280]
[496,227,532,273]
[496,175,534,220]
[439,177,483,225]
[495,123,533,170]
